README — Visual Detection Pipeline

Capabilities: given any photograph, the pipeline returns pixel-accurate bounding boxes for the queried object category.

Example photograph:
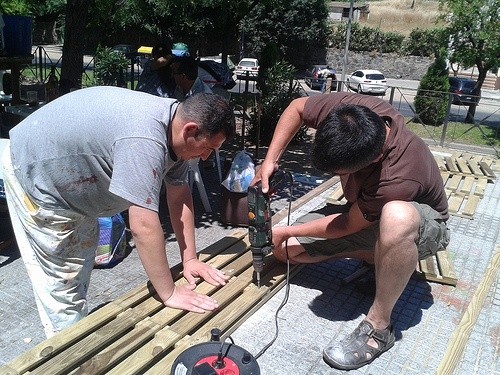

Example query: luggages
[220,192,249,226]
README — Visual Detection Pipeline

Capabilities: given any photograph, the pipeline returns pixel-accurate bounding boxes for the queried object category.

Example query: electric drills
[247,169,294,288]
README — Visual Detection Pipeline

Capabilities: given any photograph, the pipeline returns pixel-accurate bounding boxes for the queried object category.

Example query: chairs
[188,84,231,213]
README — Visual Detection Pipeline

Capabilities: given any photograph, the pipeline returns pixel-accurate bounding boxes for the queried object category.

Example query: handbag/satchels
[199,60,236,89]
[221,149,254,193]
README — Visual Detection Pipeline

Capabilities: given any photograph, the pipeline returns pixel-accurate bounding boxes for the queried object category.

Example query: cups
[27,91,37,109]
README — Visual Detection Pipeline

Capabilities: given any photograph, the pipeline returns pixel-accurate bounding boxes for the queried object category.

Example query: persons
[2,85,236,341]
[320,66,333,92]
[250,92,451,371]
[136,42,214,102]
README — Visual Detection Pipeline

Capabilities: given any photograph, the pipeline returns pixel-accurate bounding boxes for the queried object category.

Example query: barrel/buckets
[0,15,33,56]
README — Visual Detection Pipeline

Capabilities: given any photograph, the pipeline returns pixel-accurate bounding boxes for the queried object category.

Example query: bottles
[48,65,58,101]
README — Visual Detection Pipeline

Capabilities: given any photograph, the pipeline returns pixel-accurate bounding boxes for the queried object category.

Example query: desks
[4,102,48,122]
[0,55,35,125]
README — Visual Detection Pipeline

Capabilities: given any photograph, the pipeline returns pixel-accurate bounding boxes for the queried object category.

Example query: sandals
[322,318,396,371]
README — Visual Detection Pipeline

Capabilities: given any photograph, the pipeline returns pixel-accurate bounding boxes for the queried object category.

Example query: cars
[347,69,388,97]
[112,44,138,64]
[234,58,260,80]
[449,76,481,105]
[305,64,338,89]
